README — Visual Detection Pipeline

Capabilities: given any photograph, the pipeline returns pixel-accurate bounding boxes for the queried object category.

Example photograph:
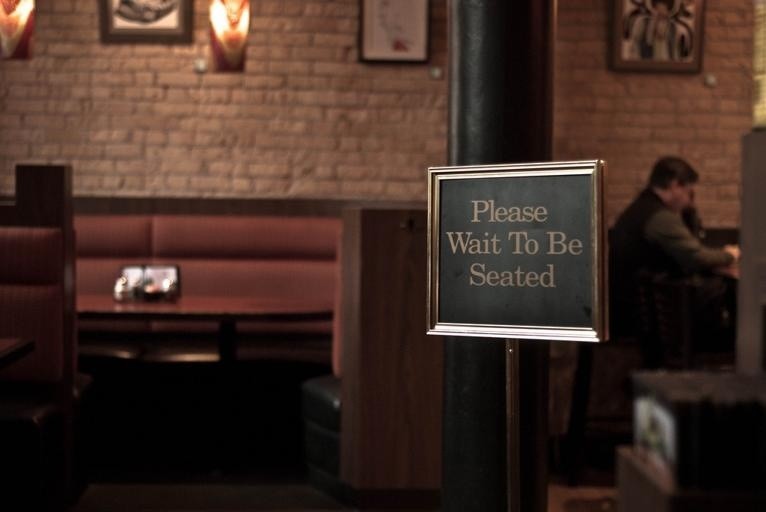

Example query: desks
[76,296,334,364]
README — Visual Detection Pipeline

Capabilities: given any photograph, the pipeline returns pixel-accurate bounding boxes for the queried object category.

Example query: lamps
[208,0,251,72]
[0,0,37,60]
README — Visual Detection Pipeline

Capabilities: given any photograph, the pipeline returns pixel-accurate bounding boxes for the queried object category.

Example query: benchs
[0,164,92,512]
[73,197,343,363]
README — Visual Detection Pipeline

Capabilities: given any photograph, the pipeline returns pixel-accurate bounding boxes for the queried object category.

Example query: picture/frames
[98,0,193,44]
[607,0,704,73]
[359,0,432,65]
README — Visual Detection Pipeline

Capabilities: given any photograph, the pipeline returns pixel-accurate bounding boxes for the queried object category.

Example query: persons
[607,154,743,365]
